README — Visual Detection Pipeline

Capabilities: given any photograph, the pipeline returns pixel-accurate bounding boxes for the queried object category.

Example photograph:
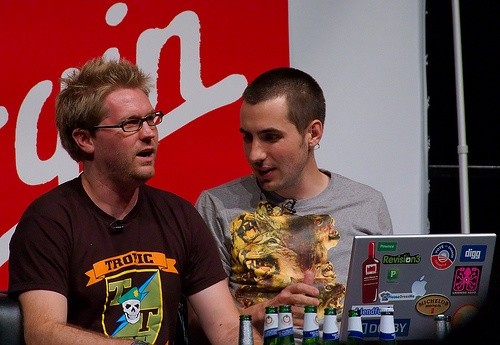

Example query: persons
[8,57,267,345]
[184,65,397,345]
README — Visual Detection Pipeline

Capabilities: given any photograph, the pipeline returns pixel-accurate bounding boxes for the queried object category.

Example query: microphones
[285,199,297,213]
[109,220,126,231]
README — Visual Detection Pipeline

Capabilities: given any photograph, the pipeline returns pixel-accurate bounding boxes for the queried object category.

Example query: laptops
[337,234,496,345]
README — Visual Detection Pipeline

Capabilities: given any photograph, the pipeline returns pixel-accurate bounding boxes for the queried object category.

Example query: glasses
[94,110,164,132]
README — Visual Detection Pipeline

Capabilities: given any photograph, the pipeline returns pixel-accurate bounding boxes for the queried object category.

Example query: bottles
[346,309,364,345]
[238,314,254,345]
[276,304,295,345]
[434,314,451,343]
[377,307,398,345]
[262,306,279,345]
[321,307,340,345]
[301,305,321,345]
[361,242,380,303]
